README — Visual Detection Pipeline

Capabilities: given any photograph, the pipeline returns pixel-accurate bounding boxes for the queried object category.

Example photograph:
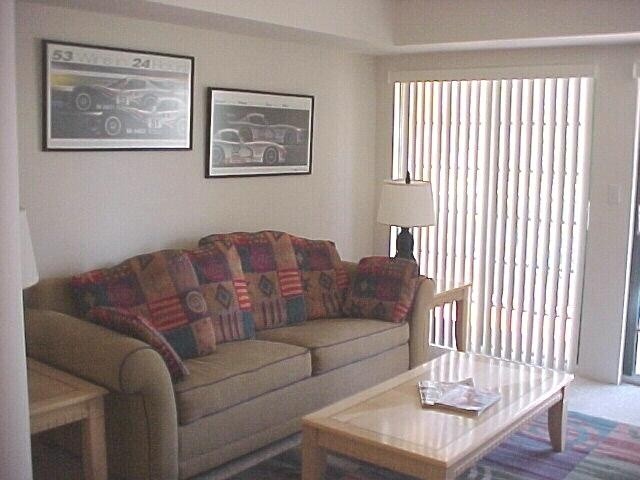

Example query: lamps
[376,170,437,262]
[19,208,40,290]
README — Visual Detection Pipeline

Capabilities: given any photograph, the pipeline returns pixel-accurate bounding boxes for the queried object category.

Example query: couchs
[23,260,436,480]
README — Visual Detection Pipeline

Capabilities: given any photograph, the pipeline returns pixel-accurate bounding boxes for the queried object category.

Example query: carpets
[218,410,640,480]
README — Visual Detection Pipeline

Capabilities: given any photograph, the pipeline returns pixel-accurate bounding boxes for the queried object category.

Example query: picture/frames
[41,38,195,152]
[205,86,314,179]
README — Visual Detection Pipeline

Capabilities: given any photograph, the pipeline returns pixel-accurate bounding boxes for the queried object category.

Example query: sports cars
[222,111,307,145]
[212,130,288,167]
[69,76,187,112]
[96,95,187,143]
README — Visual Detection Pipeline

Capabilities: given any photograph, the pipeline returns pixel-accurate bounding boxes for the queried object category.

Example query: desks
[431,278,472,352]
[27,357,111,479]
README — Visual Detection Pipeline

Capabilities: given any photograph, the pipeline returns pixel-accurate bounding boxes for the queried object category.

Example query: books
[433,383,502,418]
[417,377,477,410]
